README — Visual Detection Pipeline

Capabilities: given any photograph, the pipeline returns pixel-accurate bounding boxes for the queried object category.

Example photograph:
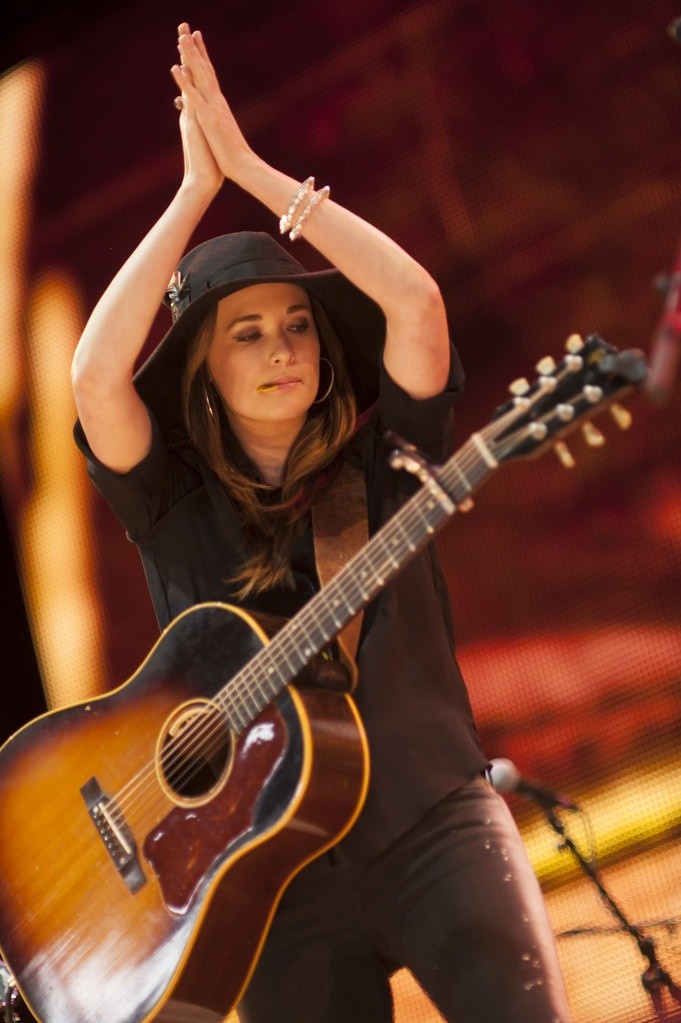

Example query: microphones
[485,758,580,812]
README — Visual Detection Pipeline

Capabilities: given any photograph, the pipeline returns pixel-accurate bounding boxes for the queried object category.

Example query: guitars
[0,330,651,1023]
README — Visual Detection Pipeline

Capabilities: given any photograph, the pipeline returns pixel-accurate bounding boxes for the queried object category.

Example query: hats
[131,231,371,388]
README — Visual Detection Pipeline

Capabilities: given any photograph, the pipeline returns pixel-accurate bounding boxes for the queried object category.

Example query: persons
[64,23,562,1023]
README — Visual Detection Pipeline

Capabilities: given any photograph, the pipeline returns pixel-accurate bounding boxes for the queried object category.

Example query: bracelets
[289,186,330,242]
[278,173,315,233]
